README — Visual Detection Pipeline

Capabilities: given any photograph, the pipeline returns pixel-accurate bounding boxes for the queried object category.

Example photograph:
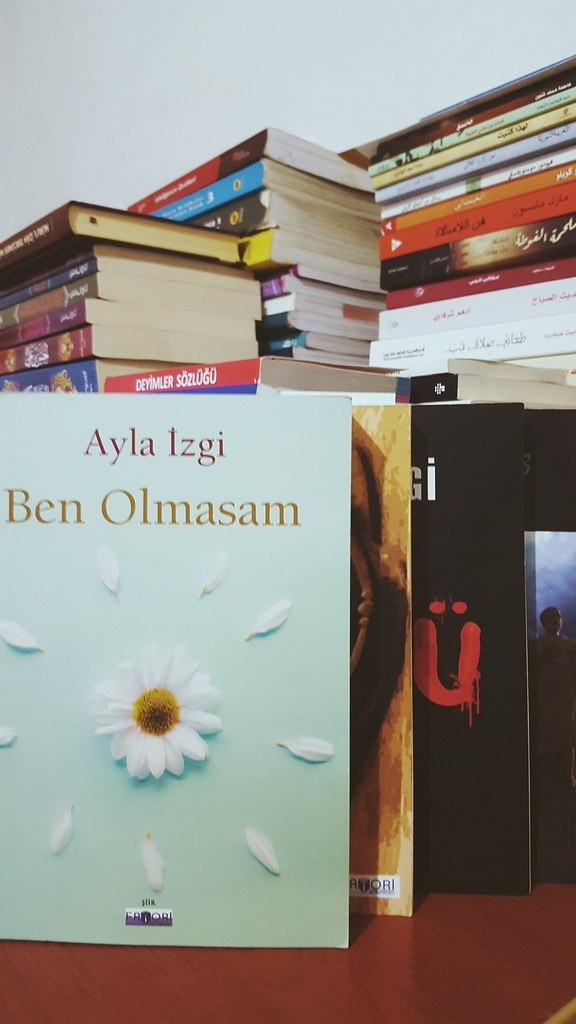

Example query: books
[0,52,576,949]
